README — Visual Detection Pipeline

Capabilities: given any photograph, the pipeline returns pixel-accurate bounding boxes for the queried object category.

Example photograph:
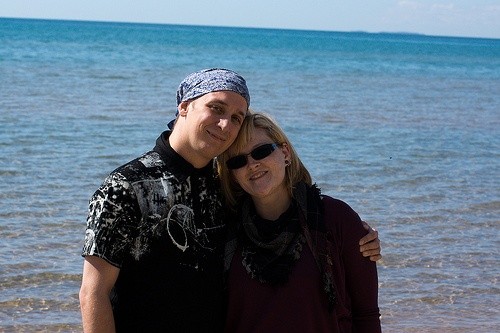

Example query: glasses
[226,142,280,170]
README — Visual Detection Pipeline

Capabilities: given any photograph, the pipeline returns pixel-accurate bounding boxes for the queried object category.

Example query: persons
[215,110,381,333]
[76,67,382,333]
